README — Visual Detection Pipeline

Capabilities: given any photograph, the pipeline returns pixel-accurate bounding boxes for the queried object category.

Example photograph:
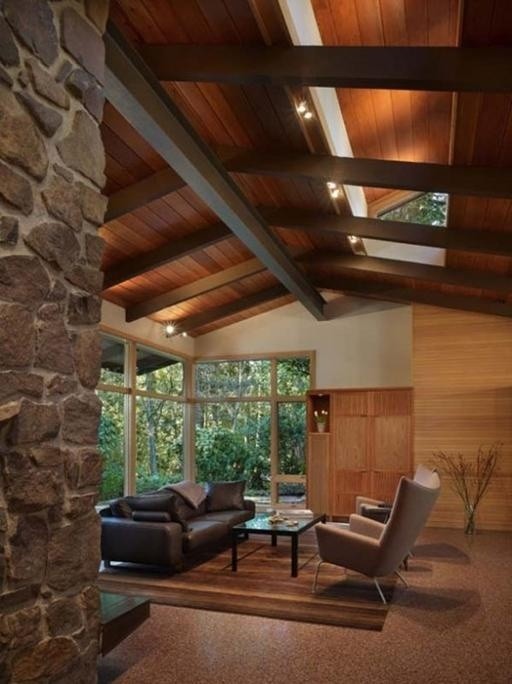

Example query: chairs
[312,463,442,605]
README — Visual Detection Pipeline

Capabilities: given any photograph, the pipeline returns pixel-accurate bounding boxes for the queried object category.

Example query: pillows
[108,480,249,533]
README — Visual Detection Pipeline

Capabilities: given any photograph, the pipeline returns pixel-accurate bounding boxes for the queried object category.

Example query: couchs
[100,494,255,576]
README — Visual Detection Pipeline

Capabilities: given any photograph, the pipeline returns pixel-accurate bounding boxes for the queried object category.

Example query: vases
[464,504,477,536]
[317,423,326,433]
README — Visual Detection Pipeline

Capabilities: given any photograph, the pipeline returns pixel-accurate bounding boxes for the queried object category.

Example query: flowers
[312,409,328,424]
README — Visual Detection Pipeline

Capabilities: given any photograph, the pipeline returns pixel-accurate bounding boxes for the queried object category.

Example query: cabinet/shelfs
[307,386,414,517]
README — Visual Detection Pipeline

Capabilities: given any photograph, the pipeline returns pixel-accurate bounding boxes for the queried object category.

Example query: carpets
[97,531,402,631]
